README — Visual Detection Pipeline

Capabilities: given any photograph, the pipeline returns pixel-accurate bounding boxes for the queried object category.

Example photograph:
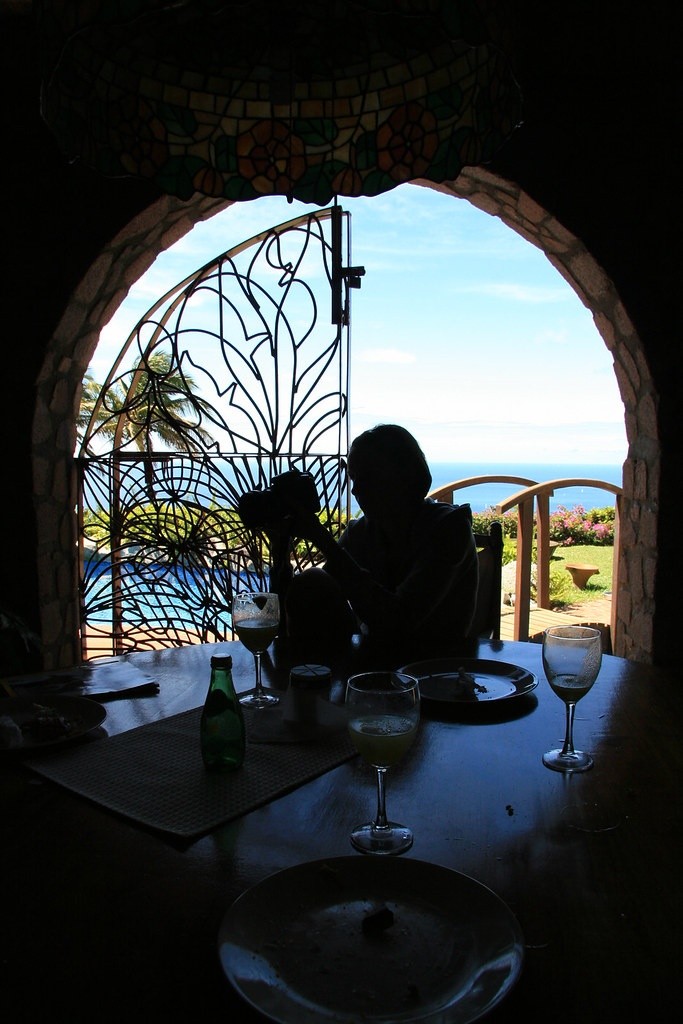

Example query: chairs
[472,521,505,640]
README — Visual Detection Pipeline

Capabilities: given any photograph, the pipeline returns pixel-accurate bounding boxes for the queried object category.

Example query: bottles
[201,654,246,773]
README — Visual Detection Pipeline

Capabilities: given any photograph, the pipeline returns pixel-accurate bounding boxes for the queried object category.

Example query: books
[0,659,160,704]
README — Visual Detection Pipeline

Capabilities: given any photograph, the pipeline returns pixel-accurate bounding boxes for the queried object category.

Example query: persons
[265,424,478,636]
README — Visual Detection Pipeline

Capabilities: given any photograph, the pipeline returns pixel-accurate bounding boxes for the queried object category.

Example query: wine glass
[543,626,603,772]
[346,671,421,854]
[231,593,281,711]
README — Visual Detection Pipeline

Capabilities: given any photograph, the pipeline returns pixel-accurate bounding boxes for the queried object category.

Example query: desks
[0,635,683,1024]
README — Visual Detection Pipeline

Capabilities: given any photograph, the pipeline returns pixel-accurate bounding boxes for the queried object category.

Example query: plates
[397,658,539,706]
[218,855,529,1024]
[0,694,108,748]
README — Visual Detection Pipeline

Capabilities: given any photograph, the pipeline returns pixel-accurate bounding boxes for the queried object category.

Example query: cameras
[238,470,321,529]
